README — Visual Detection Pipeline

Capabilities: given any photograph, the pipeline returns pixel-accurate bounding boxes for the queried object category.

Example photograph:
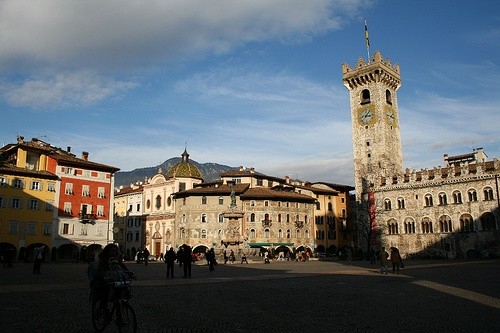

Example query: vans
[134,250,145,262]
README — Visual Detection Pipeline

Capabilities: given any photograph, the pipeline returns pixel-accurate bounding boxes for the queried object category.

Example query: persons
[378,247,389,274]
[88,244,130,315]
[2,245,57,274]
[127,244,321,279]
[370,249,377,265]
[390,247,402,274]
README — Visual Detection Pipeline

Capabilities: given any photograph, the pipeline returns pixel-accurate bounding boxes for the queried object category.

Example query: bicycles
[91,280,137,333]
